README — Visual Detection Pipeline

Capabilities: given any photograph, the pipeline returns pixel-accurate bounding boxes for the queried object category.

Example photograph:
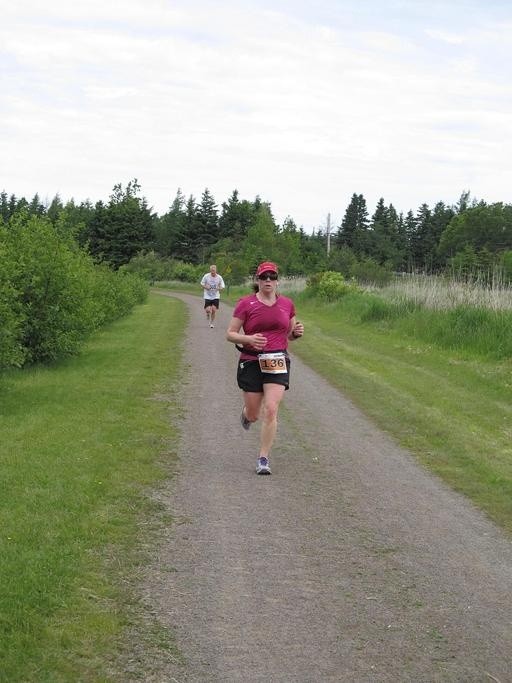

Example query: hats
[257,262,278,276]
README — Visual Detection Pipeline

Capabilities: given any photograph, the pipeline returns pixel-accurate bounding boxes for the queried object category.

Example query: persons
[201,264,224,328]
[225,261,305,479]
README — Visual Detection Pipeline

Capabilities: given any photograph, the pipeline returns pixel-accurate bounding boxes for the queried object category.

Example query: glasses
[259,272,278,280]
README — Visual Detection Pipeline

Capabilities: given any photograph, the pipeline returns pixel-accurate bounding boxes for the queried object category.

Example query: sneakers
[255,455,271,475]
[240,404,252,430]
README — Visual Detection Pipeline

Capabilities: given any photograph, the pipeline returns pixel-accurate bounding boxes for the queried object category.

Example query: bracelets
[291,329,300,338]
